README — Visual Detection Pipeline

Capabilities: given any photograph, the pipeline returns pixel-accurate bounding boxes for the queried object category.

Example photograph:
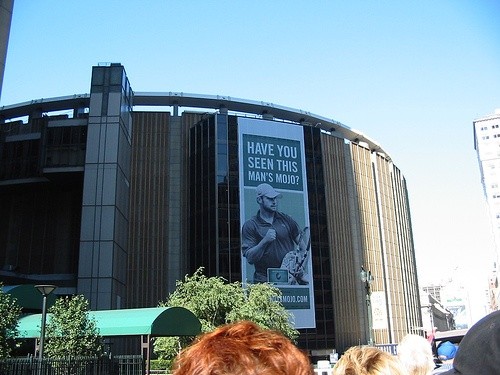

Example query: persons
[395,334,435,375]
[169,319,315,375]
[241,183,309,286]
[436,340,458,366]
[436,310,500,375]
[328,344,404,375]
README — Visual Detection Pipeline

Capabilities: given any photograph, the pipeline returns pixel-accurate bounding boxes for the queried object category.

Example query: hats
[453,309,500,373]
[257,183,281,199]
[438,340,456,360]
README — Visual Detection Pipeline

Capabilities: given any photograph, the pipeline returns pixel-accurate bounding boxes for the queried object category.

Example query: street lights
[359,265,374,349]
[34,285,56,375]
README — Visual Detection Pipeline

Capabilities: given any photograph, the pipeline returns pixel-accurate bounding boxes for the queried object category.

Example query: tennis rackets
[288,226,311,285]
